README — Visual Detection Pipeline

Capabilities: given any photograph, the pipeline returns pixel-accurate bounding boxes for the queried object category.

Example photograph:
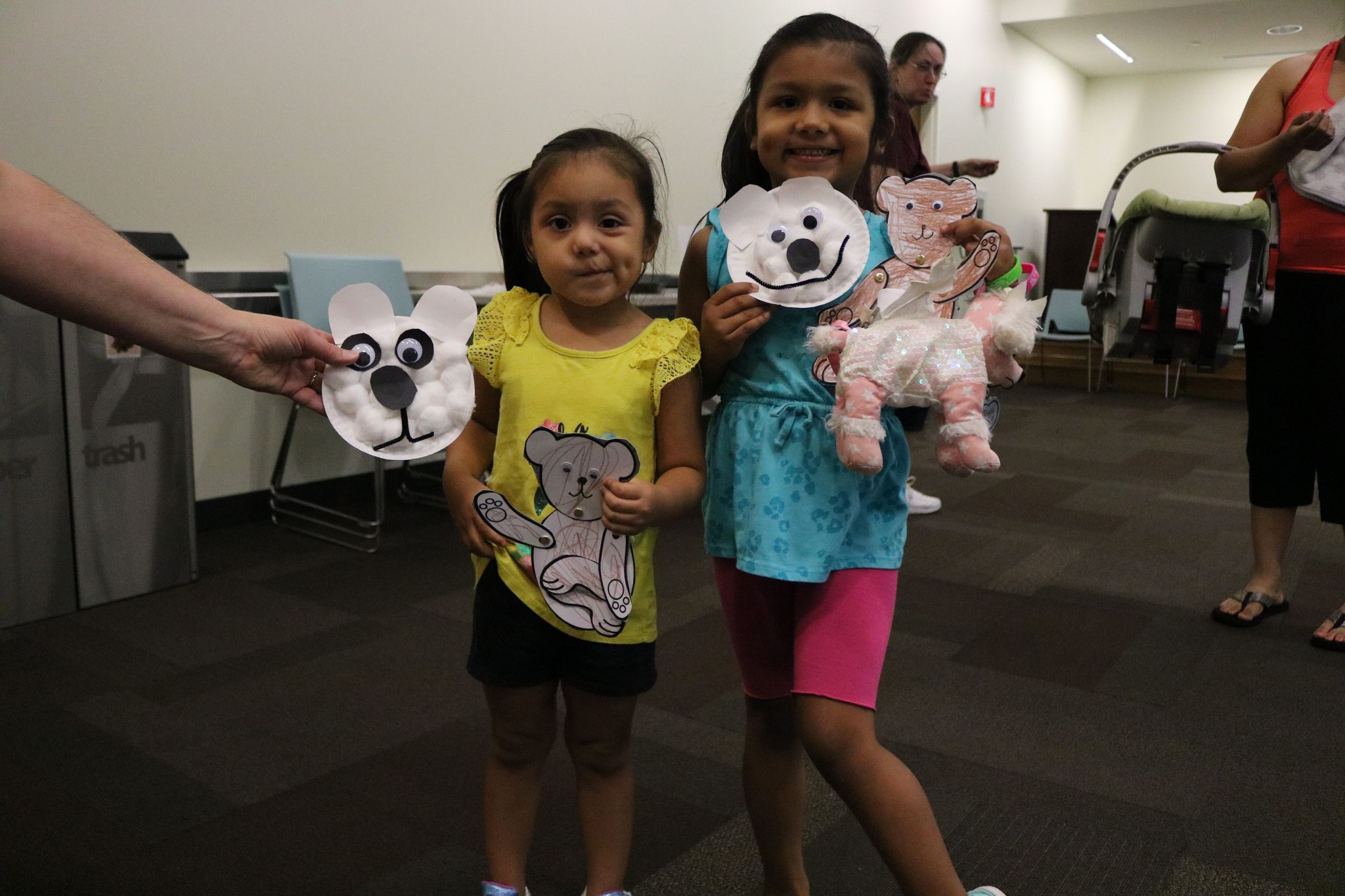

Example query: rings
[309,372,317,387]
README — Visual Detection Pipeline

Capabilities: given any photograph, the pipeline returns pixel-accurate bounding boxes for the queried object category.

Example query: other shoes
[905,476,942,514]
[966,886,1005,896]
[580,887,631,896]
[482,881,533,896]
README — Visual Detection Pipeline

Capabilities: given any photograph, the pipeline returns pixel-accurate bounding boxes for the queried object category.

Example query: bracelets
[986,254,1041,298]
[953,162,960,177]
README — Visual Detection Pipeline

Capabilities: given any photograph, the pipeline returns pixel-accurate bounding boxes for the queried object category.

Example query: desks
[411,288,678,306]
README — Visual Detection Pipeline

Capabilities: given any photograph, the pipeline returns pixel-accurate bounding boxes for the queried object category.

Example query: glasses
[902,59,948,79]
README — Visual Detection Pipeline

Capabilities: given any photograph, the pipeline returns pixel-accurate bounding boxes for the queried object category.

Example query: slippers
[1209,590,1289,626]
[1312,609,1345,652]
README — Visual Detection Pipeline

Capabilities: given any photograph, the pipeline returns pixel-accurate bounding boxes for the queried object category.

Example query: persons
[1,156,361,417]
[437,13,1024,896]
[1211,31,1345,647]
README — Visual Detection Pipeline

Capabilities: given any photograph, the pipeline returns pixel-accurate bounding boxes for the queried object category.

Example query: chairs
[269,253,451,552]
[1020,288,1092,393]
